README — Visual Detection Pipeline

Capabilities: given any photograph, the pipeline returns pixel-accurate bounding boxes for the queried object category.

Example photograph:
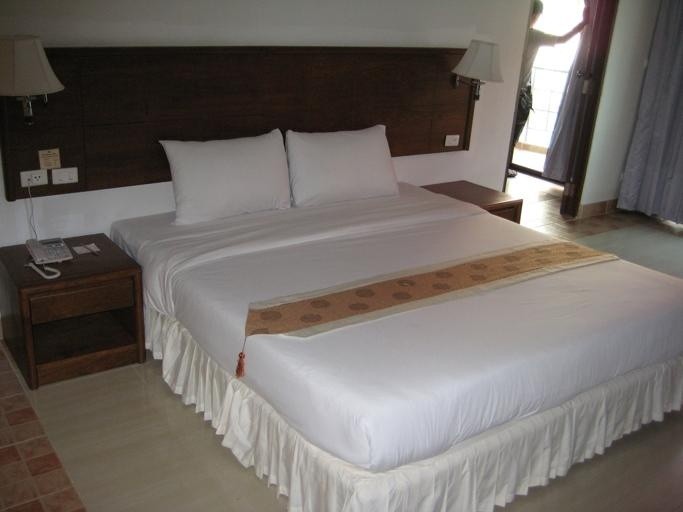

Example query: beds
[108,177,682,511]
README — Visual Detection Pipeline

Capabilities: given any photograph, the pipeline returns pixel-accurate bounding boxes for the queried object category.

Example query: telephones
[25,237,74,265]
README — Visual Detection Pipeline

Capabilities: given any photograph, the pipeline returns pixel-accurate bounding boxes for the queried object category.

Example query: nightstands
[1,233,145,390]
[421,180,524,228]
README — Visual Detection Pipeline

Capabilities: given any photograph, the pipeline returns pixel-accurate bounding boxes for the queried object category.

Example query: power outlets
[18,170,48,187]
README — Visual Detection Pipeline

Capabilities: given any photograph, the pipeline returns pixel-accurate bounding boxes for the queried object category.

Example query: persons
[507,0,587,178]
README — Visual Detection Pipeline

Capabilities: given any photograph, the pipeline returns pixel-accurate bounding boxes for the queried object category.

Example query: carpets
[0,216,682,512]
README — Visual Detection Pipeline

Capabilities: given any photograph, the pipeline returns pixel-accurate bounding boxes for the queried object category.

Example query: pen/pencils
[79,243,99,256]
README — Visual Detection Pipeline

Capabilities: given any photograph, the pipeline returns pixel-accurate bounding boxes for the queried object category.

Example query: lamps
[450,41,506,102]
[0,36,64,129]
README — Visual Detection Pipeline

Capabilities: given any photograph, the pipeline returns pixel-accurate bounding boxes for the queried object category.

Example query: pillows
[157,126,291,227]
[282,124,402,211]
